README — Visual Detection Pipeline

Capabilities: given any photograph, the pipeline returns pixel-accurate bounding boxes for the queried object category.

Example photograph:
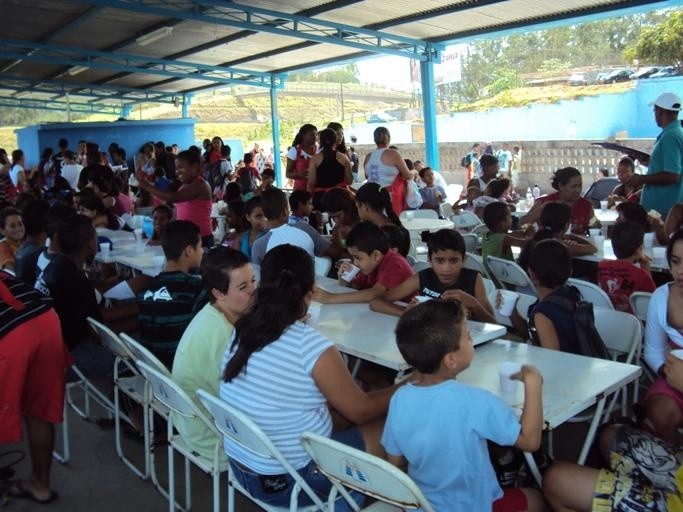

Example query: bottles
[532,185,541,199]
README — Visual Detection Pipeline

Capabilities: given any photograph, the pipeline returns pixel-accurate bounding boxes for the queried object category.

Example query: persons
[0,120,683,512]
[628,92,682,223]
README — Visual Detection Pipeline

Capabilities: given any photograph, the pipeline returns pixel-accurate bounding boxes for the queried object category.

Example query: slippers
[11,479,56,502]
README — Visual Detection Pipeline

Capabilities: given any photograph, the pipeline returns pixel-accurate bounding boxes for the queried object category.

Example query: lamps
[134,25,174,46]
[67,62,88,75]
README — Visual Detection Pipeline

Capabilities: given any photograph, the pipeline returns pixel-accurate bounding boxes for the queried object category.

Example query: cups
[154,256,164,268]
[134,229,142,240]
[601,201,608,211]
[341,265,361,282]
[643,232,654,248]
[307,301,322,324]
[499,291,518,316]
[100,243,109,255]
[218,200,224,206]
[499,362,518,394]
[406,211,413,220]
[595,237,604,251]
[519,200,525,211]
[589,229,600,237]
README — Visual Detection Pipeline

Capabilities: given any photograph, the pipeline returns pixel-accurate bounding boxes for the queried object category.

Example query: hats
[649,93,681,111]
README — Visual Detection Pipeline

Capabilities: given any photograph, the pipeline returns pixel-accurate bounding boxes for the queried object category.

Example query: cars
[562,63,680,82]
